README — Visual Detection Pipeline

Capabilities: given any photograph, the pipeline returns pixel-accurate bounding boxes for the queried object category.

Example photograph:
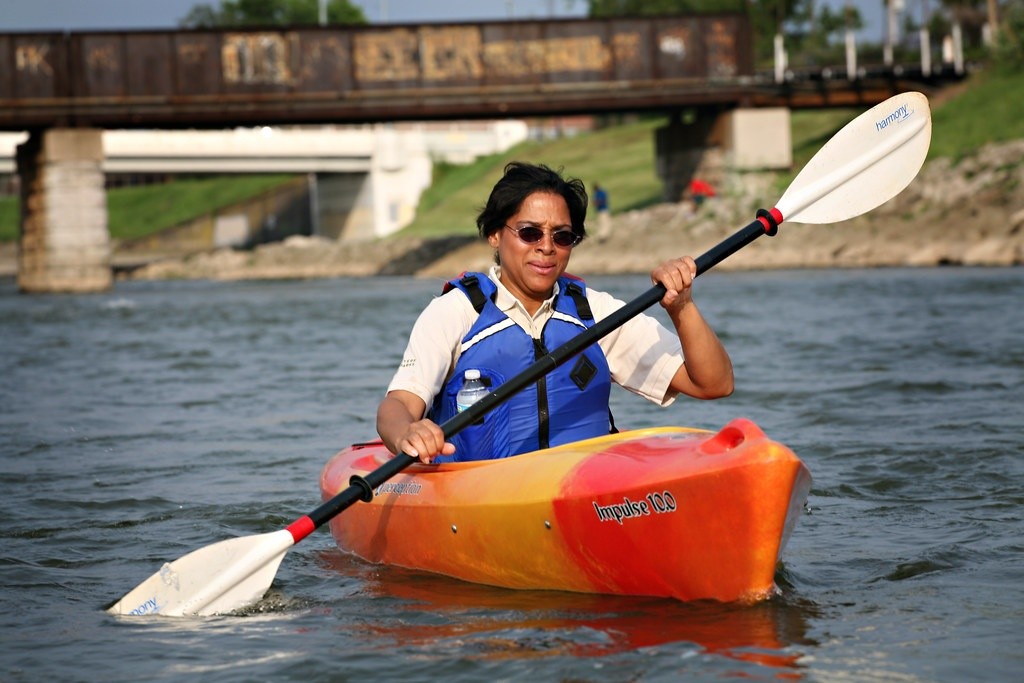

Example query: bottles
[457,370,490,413]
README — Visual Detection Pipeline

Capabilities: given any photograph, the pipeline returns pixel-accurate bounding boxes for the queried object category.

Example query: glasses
[505,224,580,249]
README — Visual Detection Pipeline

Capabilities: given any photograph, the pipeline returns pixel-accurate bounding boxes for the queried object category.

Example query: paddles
[101,87,934,620]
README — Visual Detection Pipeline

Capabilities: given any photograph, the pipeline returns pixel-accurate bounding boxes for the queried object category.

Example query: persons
[376,161,735,464]
[592,182,610,243]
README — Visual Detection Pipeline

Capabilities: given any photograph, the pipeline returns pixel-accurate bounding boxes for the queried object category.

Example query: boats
[319,415,815,613]
